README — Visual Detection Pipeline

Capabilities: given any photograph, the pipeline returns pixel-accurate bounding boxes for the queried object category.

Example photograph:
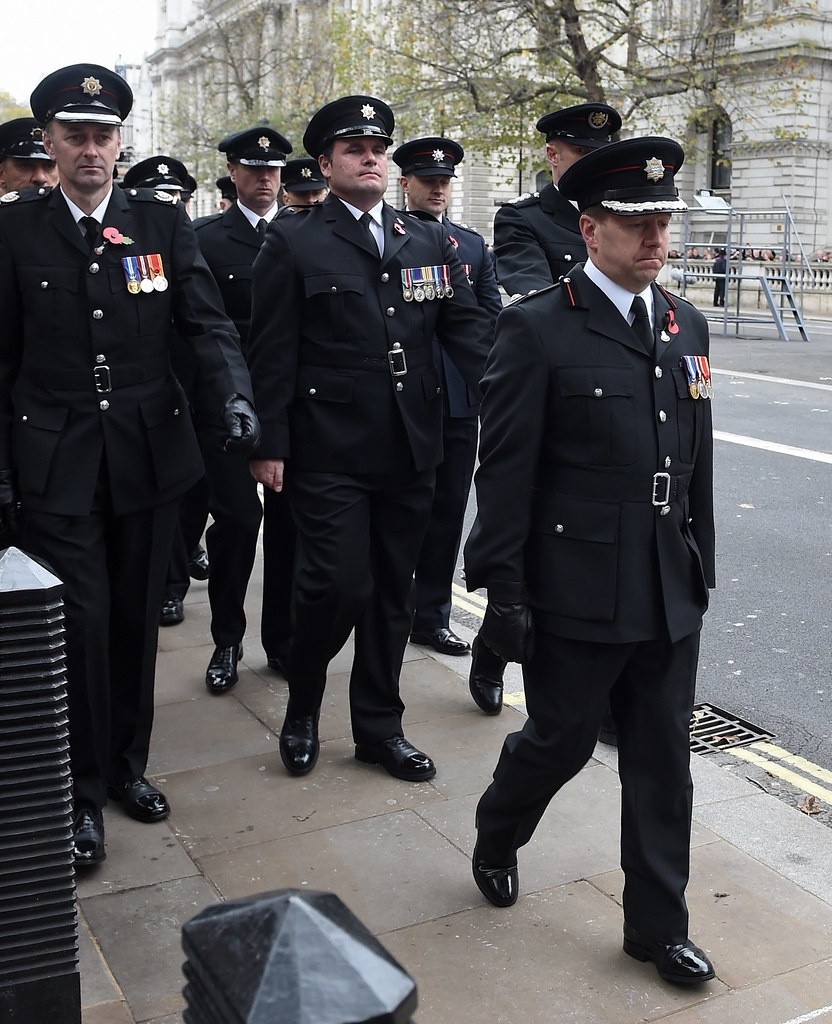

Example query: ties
[258,218,268,241]
[360,212,382,261]
[629,295,655,359]
[80,216,99,250]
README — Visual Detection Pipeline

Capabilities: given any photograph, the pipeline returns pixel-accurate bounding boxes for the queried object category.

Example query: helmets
[719,248,726,255]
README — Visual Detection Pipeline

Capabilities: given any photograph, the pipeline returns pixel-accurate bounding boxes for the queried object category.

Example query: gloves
[0,469,19,538]
[214,392,263,461]
[479,579,536,665]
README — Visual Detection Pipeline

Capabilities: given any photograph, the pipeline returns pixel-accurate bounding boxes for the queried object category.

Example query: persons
[0,96,501,781]
[664,243,832,295]
[461,135,716,983]
[711,250,727,307]
[451,103,623,746]
[0,64,263,872]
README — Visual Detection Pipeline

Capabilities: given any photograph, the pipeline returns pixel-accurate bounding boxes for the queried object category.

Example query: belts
[544,472,694,506]
[299,344,434,378]
[23,351,172,394]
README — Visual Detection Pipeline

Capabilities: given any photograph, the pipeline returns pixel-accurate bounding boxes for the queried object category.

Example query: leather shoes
[190,543,210,581]
[622,930,717,983]
[102,774,170,825]
[205,640,244,693]
[469,635,508,715]
[160,597,185,625]
[72,805,107,867]
[470,802,519,908]
[353,733,437,783]
[409,626,471,655]
[279,699,321,778]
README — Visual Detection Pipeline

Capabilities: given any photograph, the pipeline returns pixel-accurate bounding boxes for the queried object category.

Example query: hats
[558,136,691,216]
[745,243,751,246]
[0,117,54,161]
[30,62,134,126]
[118,155,198,199]
[218,126,293,167]
[536,102,622,149]
[280,159,328,191]
[394,136,464,178]
[216,176,238,198]
[303,95,395,161]
